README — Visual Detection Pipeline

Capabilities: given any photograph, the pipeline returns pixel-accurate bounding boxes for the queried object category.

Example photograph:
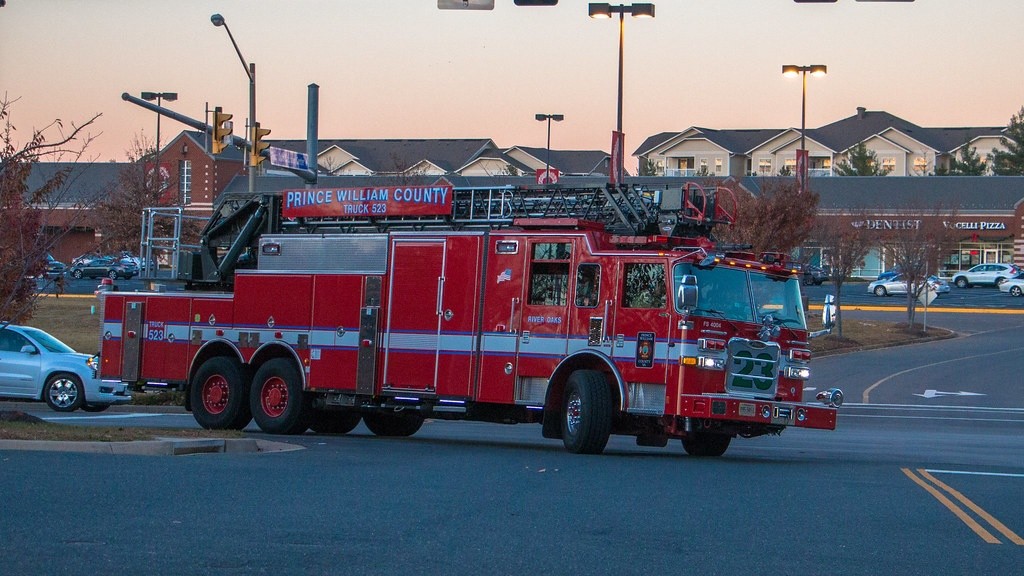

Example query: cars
[0,324,132,412]
[878,272,898,282]
[998,271,1024,297]
[35,253,66,280]
[72,253,98,267]
[803,264,828,286]
[69,258,139,280]
[867,272,950,299]
[136,257,154,271]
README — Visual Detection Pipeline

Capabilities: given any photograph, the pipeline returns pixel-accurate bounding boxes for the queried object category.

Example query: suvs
[950,262,1021,288]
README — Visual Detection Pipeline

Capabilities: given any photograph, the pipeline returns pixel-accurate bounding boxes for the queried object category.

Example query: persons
[630,277,667,308]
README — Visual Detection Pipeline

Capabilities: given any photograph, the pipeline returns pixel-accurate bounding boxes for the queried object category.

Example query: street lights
[141,92,178,204]
[536,114,564,183]
[211,14,256,192]
[588,3,655,182]
[782,64,827,194]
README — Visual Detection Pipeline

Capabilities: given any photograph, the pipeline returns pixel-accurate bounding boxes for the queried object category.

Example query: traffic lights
[212,107,233,155]
[250,127,271,165]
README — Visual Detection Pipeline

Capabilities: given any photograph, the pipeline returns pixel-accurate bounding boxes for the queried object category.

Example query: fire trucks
[99,182,844,455]
